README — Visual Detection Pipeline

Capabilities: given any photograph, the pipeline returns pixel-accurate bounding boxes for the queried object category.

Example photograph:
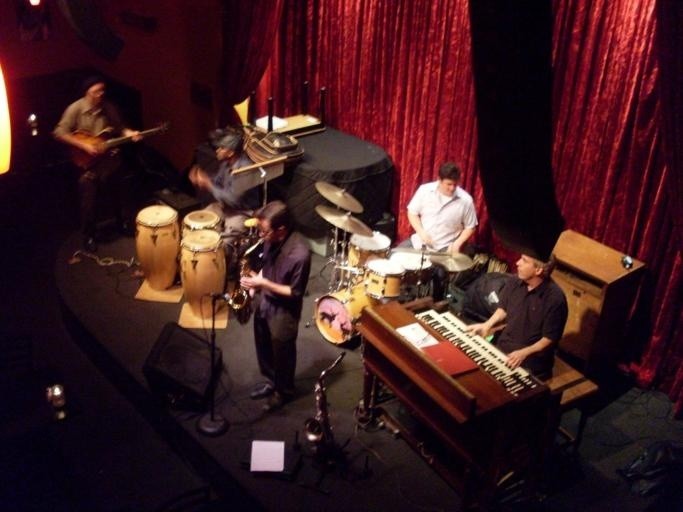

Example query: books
[247,437,288,476]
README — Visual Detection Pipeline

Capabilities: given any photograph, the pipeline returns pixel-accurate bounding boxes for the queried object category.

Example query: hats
[216,135,239,151]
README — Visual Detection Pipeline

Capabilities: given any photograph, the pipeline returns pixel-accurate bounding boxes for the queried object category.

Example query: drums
[361,259,406,300]
[387,248,434,286]
[314,283,372,347]
[347,232,391,268]
[136,205,178,291]
[180,232,225,317]
[182,210,222,231]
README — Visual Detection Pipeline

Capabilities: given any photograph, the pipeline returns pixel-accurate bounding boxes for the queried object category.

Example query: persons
[50,76,142,267]
[186,132,268,289]
[238,201,310,417]
[452,267,532,336]
[392,161,480,281]
[461,239,570,386]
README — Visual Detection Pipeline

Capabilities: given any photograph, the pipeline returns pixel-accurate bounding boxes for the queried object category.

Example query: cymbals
[429,252,473,271]
[316,205,373,237]
[316,180,364,214]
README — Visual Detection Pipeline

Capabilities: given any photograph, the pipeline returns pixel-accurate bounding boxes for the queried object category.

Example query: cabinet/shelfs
[546,230,648,371]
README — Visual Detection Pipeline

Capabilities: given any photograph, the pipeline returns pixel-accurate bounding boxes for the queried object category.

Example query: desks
[247,80,326,140]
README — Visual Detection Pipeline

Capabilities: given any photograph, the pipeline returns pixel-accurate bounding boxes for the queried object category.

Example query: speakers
[144,322,223,414]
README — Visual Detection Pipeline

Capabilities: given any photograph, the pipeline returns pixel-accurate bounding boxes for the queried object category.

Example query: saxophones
[303,352,346,447]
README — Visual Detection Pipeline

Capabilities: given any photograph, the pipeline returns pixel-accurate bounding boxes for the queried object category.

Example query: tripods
[305,205,350,327]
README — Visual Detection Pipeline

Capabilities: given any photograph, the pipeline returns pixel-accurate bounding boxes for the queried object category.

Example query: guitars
[72,121,174,168]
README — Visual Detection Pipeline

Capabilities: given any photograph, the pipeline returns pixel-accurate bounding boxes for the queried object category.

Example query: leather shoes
[262,392,284,412]
[249,383,273,400]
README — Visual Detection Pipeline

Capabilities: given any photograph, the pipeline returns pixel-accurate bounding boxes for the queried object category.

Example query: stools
[539,356,598,451]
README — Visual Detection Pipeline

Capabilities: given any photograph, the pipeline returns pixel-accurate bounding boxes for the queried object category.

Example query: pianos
[356,298,562,498]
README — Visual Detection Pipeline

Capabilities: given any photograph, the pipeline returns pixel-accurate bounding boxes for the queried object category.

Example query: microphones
[206,293,230,301]
